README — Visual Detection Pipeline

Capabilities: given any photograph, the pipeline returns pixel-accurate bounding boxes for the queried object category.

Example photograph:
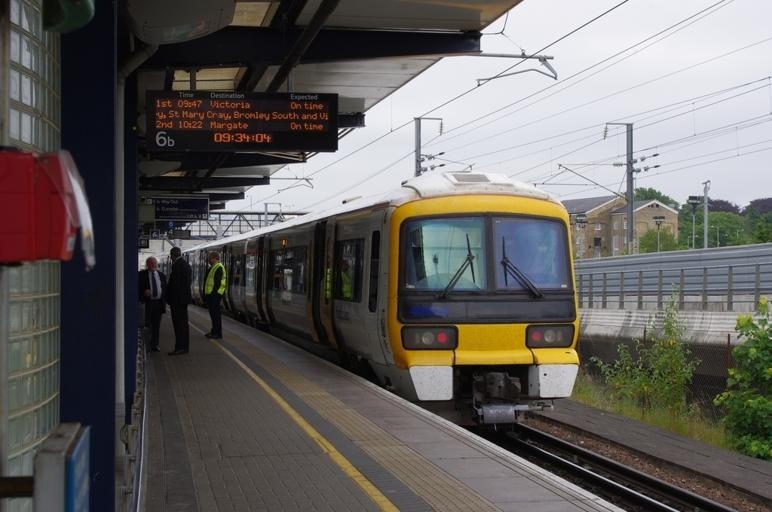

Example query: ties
[151,272,158,299]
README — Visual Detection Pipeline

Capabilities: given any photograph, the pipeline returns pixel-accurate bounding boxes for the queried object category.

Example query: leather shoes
[167,348,190,356]
[204,332,223,338]
[153,344,161,352]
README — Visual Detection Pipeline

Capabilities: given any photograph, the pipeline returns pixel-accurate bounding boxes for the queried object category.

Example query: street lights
[594,237,601,261]
[688,180,712,248]
[653,215,666,253]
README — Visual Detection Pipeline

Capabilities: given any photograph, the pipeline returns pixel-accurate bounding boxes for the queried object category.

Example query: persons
[324,259,333,299]
[165,247,192,356]
[139,256,168,353]
[203,251,228,338]
[340,259,351,300]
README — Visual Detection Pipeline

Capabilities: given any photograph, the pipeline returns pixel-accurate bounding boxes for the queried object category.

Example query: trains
[159,171,580,433]
